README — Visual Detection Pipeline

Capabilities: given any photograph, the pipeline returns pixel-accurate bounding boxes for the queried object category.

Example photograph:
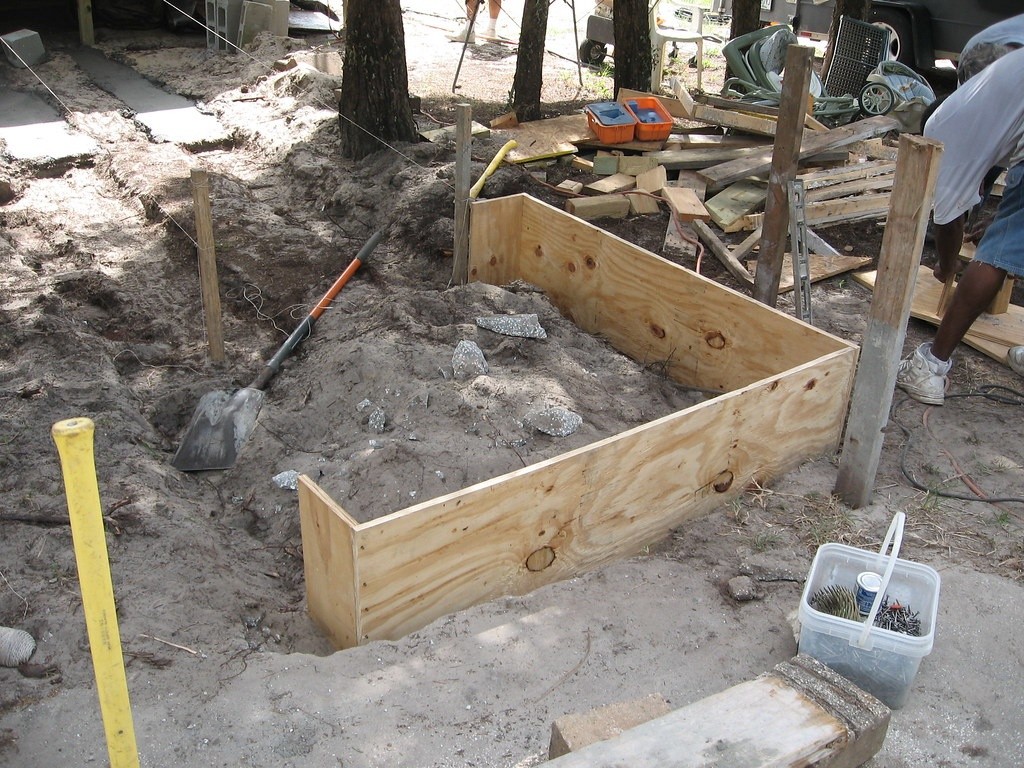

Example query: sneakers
[1007,347,1024,377]
[896,342,951,405]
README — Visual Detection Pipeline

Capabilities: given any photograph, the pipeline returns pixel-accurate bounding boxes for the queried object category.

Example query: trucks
[713,0,1024,77]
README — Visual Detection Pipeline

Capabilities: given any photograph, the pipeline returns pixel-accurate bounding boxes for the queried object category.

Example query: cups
[852,572,885,615]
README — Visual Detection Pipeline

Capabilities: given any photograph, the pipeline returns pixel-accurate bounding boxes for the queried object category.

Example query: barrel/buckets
[798,511,941,710]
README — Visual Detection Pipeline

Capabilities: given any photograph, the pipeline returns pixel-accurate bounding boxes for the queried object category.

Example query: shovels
[172,229,383,470]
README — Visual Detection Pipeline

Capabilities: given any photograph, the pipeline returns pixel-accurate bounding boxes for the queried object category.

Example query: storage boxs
[623,97,673,143]
[584,103,636,144]
[824,15,890,99]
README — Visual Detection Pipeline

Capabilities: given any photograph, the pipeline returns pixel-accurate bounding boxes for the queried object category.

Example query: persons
[895,45,1024,406]
[445,0,501,43]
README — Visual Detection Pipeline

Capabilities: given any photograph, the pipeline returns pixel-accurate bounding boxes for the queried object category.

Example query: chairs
[649,6,702,91]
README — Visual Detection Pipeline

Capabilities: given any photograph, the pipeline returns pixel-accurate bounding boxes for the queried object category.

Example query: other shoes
[487,28,495,37]
[451,30,475,43]
